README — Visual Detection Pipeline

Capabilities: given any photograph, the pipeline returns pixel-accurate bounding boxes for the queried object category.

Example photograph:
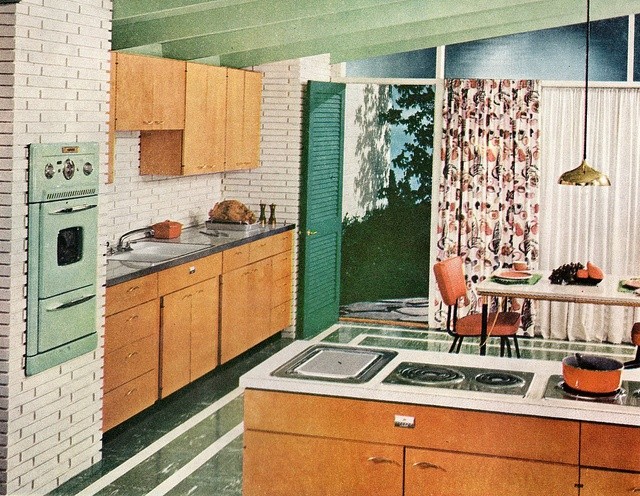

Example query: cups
[512,261,528,270]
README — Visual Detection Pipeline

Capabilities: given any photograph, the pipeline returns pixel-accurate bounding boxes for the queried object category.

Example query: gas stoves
[381,361,535,400]
[544,373,640,409]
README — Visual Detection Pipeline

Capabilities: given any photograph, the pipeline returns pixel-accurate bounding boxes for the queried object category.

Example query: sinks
[108,242,214,262]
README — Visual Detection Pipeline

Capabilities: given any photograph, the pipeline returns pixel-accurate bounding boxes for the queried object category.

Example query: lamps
[557,0,613,189]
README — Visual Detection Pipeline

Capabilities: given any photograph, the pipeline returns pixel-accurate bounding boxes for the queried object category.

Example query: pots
[147,218,186,241]
[563,351,627,394]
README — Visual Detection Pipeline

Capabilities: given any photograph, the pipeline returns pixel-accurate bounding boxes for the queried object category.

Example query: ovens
[29,142,99,375]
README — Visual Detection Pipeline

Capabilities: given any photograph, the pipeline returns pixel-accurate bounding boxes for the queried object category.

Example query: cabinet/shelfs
[138,58,263,178]
[105,51,185,183]
[102,237,224,437]
[142,217,292,368]
[238,337,640,496]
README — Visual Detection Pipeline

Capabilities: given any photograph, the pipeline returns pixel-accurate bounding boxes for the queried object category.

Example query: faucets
[119,228,155,252]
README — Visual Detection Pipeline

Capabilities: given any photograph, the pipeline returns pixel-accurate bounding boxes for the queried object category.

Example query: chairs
[433,255,523,359]
[620,323,640,370]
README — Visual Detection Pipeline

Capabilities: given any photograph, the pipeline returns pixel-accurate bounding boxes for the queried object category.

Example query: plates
[494,270,533,280]
[623,280,640,289]
